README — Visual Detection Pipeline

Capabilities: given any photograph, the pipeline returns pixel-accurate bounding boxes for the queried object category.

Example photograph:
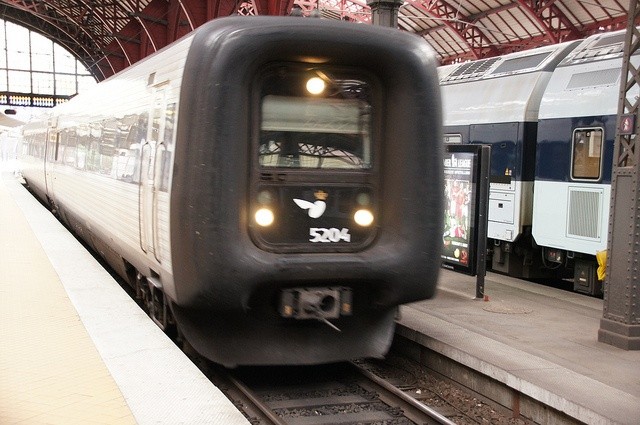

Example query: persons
[443,179,471,240]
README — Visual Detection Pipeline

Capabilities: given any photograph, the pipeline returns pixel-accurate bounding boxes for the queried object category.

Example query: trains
[0,15,445,366]
[431,26,640,294]
